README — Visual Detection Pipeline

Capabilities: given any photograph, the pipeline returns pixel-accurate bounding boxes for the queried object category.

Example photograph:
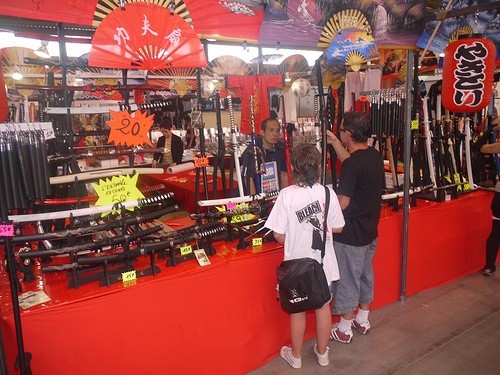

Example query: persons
[264,142,345,369]
[236,117,288,195]
[319,111,386,344]
[472,114,500,276]
[152,119,183,166]
[84,115,95,145]
[73,117,87,153]
[184,115,196,148]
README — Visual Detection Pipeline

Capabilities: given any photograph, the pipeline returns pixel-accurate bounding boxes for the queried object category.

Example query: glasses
[338,126,346,133]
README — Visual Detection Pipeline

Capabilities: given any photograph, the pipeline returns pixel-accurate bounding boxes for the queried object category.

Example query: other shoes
[482,263,496,276]
[313,343,330,366]
[280,345,302,369]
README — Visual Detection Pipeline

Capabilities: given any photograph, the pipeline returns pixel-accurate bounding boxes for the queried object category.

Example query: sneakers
[350,315,371,335]
[329,322,354,344]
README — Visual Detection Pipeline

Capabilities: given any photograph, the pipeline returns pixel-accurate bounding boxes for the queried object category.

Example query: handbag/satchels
[277,257,331,314]
